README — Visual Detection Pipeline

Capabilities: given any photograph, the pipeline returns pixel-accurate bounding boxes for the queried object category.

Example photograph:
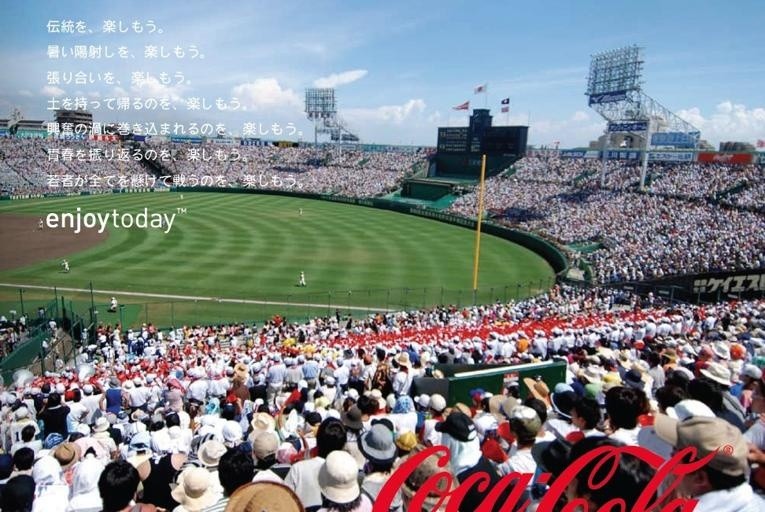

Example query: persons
[0,136,765,512]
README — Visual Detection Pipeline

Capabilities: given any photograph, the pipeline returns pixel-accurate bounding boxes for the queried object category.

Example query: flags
[474,84,487,94]
[501,106,509,112]
[452,101,469,110]
[501,98,510,104]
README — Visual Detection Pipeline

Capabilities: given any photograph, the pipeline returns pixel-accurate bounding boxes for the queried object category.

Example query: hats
[1,281,764,511]
